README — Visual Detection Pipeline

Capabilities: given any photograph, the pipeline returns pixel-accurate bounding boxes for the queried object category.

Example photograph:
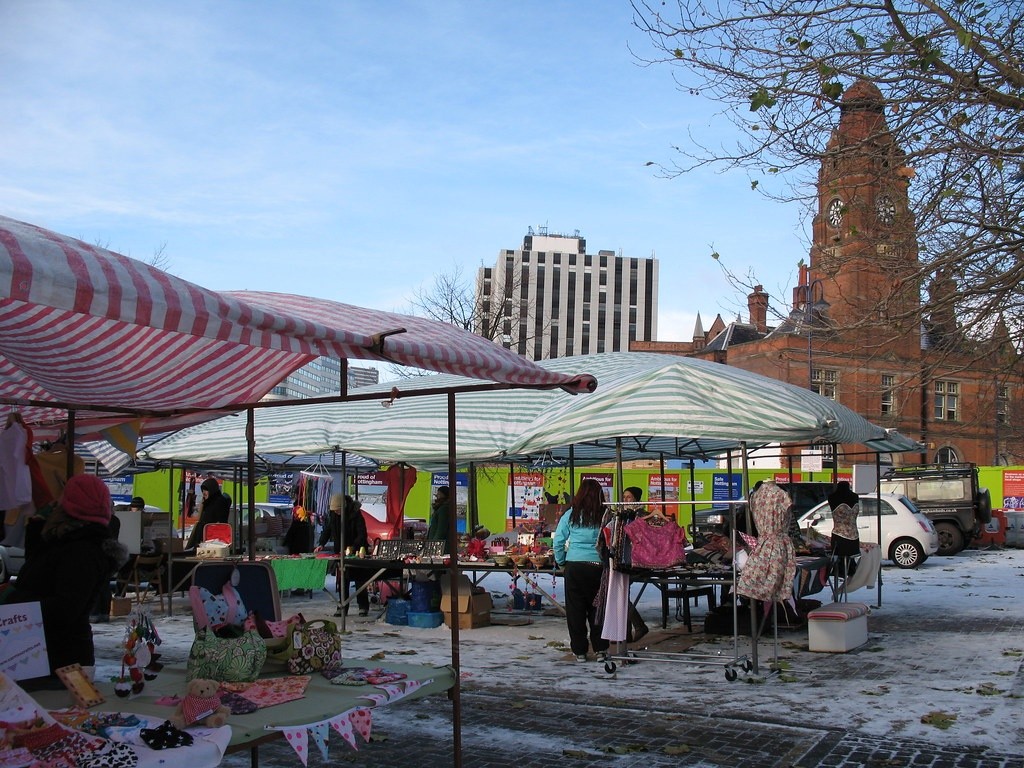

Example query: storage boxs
[441,592,493,629]
[197,523,234,558]
[0,524,894,768]
[109,596,131,617]
[406,609,443,628]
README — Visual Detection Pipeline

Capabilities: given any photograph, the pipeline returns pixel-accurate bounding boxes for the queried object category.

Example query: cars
[0,501,179,598]
[360,502,428,543]
[798,493,939,569]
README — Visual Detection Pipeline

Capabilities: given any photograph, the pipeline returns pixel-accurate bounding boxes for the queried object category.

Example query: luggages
[703,604,770,635]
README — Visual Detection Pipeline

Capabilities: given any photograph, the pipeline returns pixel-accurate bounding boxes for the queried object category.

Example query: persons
[827,481,860,576]
[116,497,145,596]
[313,495,370,616]
[184,478,232,552]
[426,486,451,578]
[0,475,113,671]
[736,476,797,605]
[552,479,610,661]
[614,487,649,642]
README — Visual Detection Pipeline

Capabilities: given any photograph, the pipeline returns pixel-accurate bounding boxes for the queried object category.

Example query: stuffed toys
[166,679,231,730]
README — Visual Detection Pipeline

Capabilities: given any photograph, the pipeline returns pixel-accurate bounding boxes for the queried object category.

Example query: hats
[60,474,111,523]
[130,497,144,508]
[329,494,346,510]
[624,487,643,502]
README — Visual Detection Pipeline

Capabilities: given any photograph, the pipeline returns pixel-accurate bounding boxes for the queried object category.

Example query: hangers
[299,453,332,479]
[5,395,65,449]
[607,501,670,523]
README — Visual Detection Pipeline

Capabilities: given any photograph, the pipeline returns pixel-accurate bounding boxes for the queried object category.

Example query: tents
[0,211,597,766]
[20,346,935,668]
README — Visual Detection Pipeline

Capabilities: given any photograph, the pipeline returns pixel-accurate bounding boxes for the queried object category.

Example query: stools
[808,602,869,651]
[663,587,716,631]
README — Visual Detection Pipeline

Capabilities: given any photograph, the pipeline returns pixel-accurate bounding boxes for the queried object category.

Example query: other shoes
[595,650,607,662]
[572,653,588,662]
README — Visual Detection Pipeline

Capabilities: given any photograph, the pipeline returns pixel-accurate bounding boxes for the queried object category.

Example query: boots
[610,621,633,644]
[630,609,649,642]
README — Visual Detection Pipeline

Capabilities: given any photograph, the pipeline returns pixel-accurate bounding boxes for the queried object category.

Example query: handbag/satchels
[687,531,739,565]
[290,620,342,677]
[185,618,267,684]
[246,612,307,662]
[190,579,248,632]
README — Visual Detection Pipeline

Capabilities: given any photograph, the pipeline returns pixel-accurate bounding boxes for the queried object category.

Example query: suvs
[873,460,994,557]
[177,503,300,556]
[685,480,841,539]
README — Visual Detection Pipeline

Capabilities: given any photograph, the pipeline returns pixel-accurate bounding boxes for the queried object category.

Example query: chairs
[193,562,281,641]
[119,555,166,617]
